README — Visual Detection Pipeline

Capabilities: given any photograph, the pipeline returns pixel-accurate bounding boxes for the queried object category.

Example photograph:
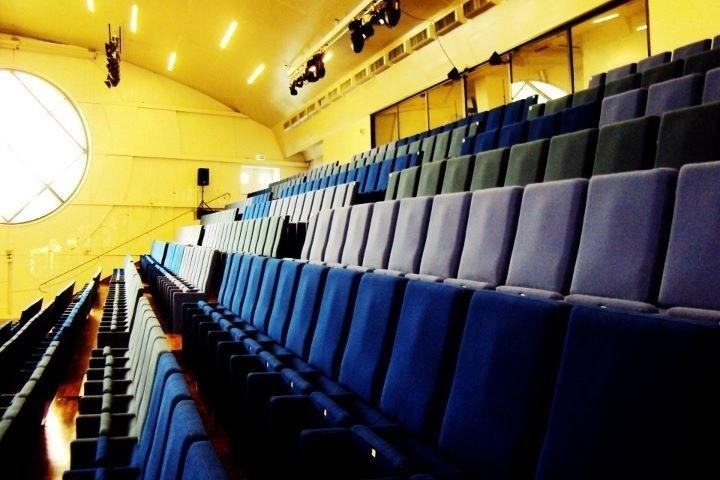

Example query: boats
[197,168,209,186]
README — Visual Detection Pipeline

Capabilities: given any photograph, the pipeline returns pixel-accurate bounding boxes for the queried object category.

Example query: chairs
[1,33,720,480]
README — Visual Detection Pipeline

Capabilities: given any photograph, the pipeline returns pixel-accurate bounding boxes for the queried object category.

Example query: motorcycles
[197,186,213,212]
